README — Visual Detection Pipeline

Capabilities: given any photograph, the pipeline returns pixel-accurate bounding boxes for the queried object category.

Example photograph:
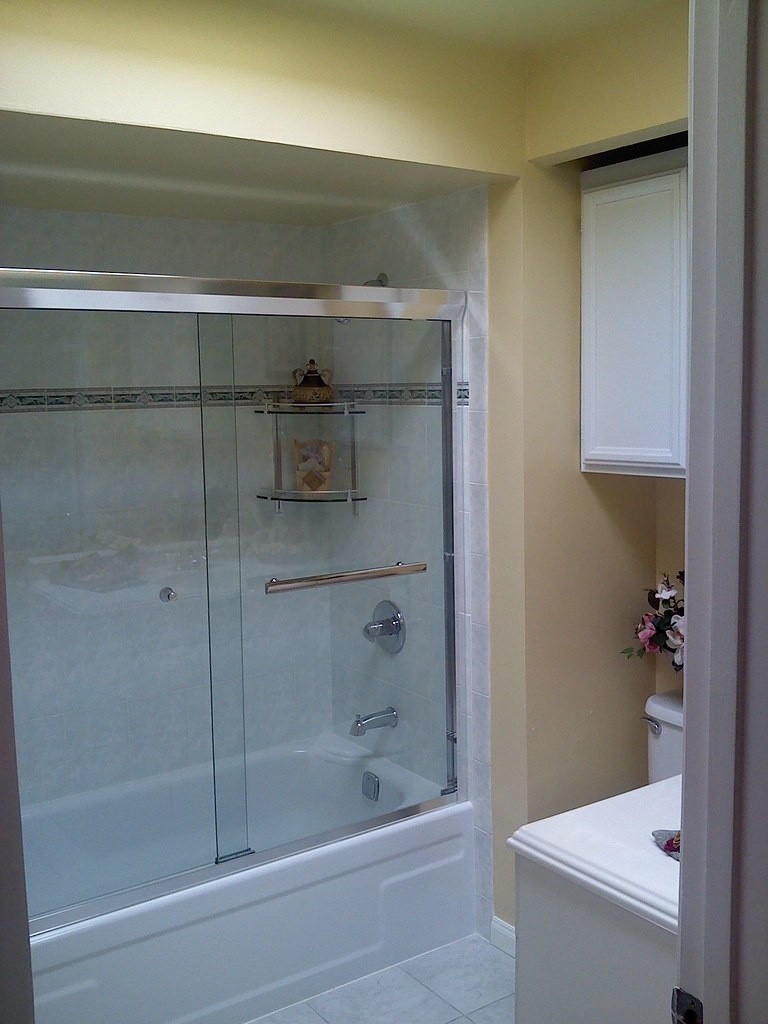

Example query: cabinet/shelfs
[578,166,688,480]
[503,771,681,1024]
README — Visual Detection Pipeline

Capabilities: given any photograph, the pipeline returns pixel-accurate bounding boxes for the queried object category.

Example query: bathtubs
[18,729,477,1024]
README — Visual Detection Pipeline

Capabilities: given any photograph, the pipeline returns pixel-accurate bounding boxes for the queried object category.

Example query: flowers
[619,571,685,671]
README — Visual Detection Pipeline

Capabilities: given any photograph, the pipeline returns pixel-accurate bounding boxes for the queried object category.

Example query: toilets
[638,695,687,789]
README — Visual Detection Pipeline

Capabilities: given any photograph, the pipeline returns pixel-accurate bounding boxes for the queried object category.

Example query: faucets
[348,706,400,738]
